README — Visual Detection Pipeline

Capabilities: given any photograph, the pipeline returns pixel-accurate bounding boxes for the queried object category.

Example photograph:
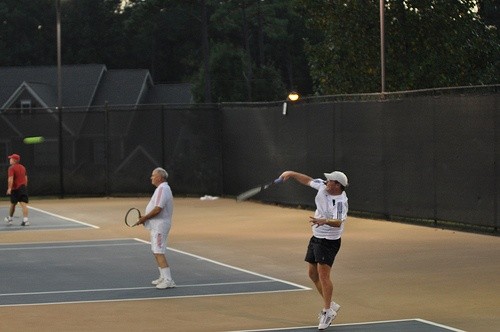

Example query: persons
[138,168,176,289]
[279,170,349,330]
[4,154,29,226]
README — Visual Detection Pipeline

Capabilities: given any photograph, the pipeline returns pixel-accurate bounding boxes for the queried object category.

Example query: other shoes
[5,217,13,224]
[21,222,28,226]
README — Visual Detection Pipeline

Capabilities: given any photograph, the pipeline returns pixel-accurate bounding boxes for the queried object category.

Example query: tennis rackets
[125,207,146,228]
[236,177,282,201]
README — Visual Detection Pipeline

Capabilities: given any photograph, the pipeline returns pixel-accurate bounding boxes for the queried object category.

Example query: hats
[8,154,20,160]
[324,171,347,187]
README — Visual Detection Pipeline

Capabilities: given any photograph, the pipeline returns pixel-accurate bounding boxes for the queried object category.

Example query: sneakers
[323,302,341,314]
[318,309,336,329]
[156,280,176,288]
[152,278,165,285]
